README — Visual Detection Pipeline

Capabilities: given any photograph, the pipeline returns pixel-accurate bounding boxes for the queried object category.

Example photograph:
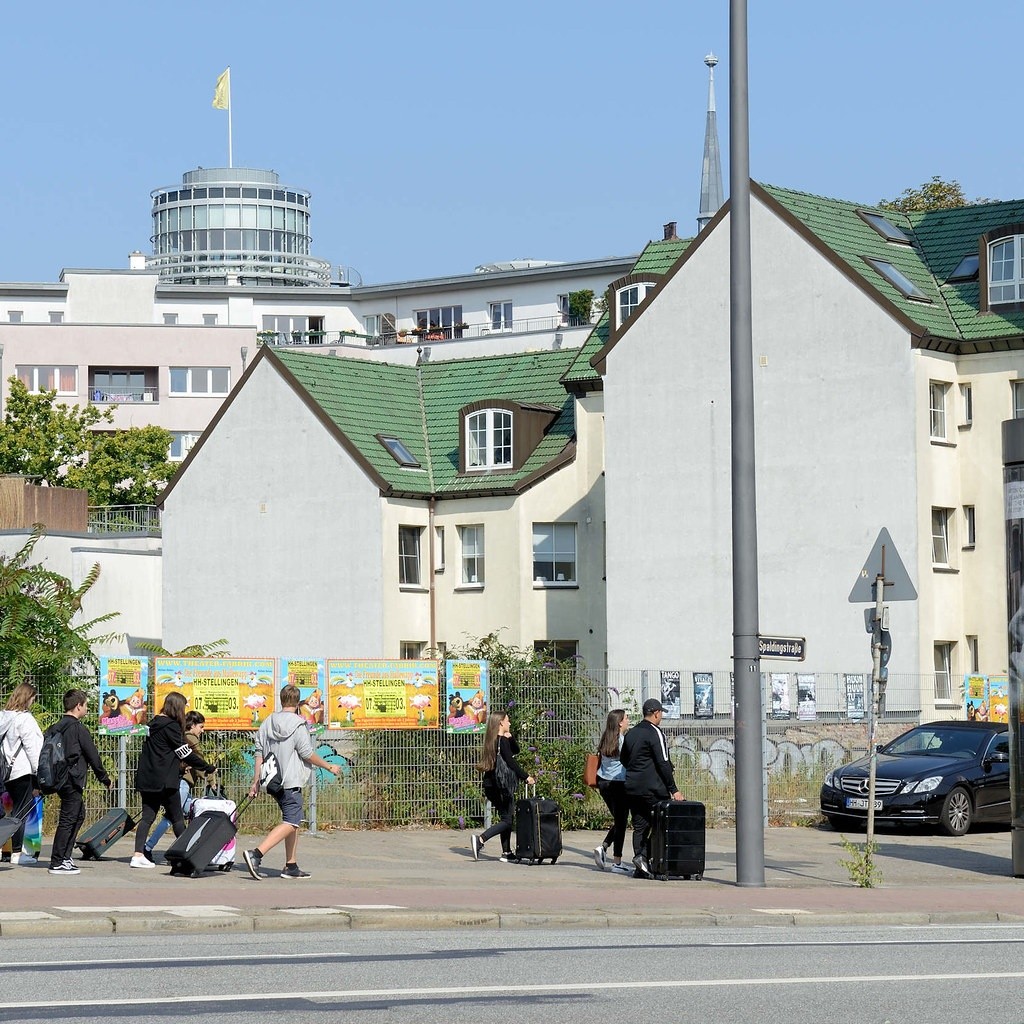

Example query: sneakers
[471,835,484,860]
[633,854,654,879]
[611,861,633,875]
[594,846,607,869]
[243,850,263,880]
[9,851,37,865]
[500,851,521,862]
[48,856,80,875]
[142,845,156,864]
[130,854,157,868]
[280,867,311,879]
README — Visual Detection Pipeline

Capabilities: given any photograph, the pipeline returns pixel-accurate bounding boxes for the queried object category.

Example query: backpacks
[37,718,80,794]
[259,752,294,800]
[0,730,23,789]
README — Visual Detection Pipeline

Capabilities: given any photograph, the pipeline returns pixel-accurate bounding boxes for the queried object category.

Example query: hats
[643,699,668,715]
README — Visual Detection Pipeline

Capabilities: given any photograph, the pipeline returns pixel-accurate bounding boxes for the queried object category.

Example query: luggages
[188,770,237,871]
[1,791,44,861]
[164,793,257,878]
[649,798,705,881]
[514,780,562,865]
[73,808,143,861]
[0,792,45,849]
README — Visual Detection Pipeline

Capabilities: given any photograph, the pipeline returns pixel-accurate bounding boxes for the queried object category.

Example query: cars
[820,720,1024,837]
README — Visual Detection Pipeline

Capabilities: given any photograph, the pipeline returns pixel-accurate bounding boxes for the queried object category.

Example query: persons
[621,699,686,877]
[43,689,111,874]
[593,709,634,875]
[242,686,340,880]
[130,693,218,868]
[470,711,535,862]
[0,683,43,864]
[144,711,213,863]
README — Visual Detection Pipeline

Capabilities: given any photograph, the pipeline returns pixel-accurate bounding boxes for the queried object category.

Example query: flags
[213,70,229,109]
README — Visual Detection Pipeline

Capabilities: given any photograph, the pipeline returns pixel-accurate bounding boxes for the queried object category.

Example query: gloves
[102,778,111,786]
[207,766,216,774]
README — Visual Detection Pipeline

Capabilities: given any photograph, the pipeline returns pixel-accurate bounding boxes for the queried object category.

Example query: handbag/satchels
[495,735,517,797]
[582,738,605,787]
